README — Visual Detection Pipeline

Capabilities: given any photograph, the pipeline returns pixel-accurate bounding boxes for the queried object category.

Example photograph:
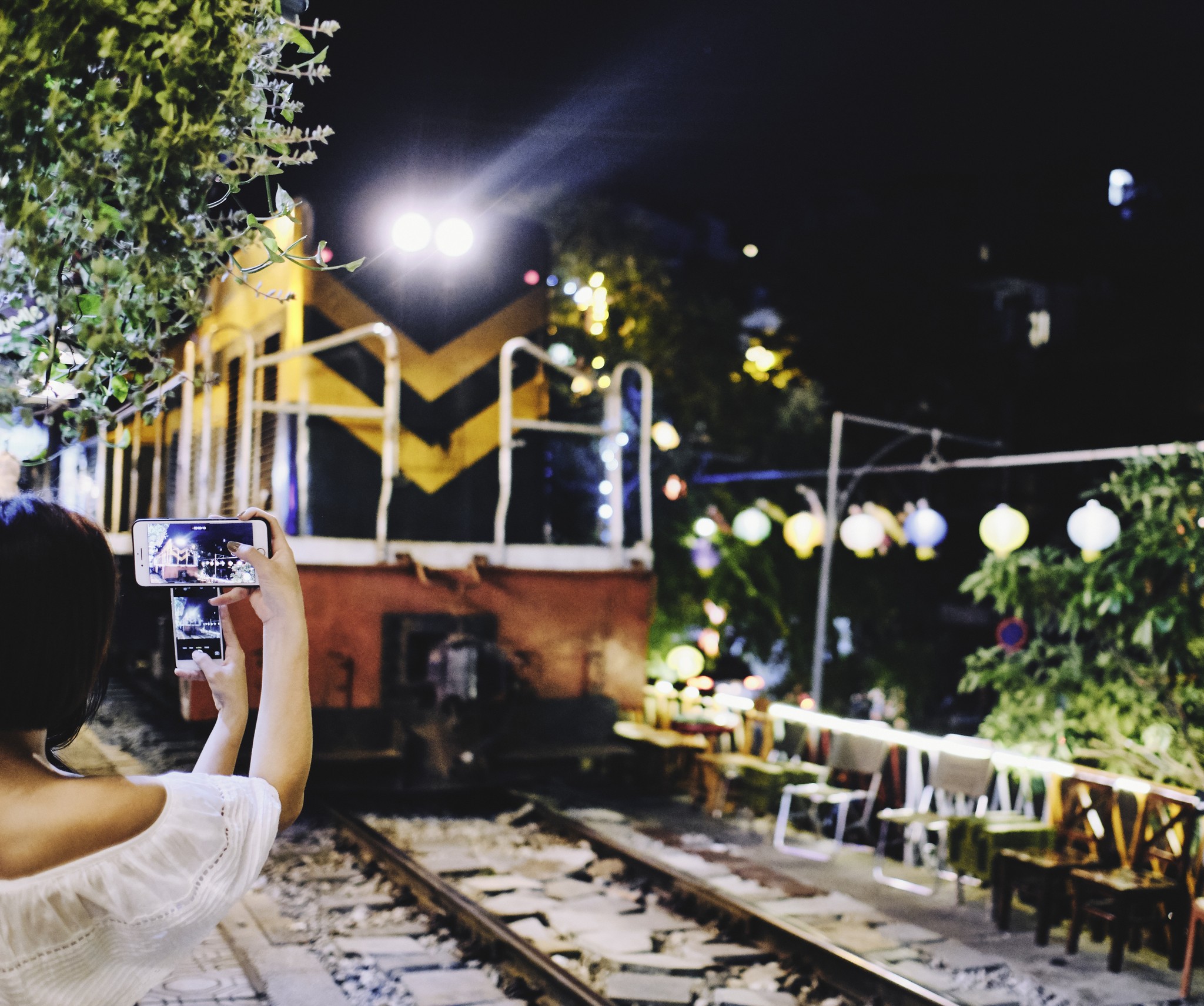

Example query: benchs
[455,695,635,809]
[236,708,406,815]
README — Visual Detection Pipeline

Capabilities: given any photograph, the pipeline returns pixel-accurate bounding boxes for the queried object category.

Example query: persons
[175,602,251,777]
[0,494,315,1006]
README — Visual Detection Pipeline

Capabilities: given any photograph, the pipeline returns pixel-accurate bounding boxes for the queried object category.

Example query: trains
[10,191,663,805]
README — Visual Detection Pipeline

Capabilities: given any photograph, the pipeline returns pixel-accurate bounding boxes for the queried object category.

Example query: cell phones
[170,587,226,669]
[132,516,273,588]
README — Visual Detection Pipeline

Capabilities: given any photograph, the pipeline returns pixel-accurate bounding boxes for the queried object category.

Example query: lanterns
[731,506,772,547]
[782,510,823,559]
[978,502,1030,563]
[904,506,947,561]
[1067,498,1120,564]
[838,512,884,558]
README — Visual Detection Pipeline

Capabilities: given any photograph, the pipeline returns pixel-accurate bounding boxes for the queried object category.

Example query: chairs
[872,752,1048,920]
[992,776,1137,946]
[773,733,889,861]
[1181,835,1204,1006]
[614,690,822,837]
[1072,790,1197,972]
